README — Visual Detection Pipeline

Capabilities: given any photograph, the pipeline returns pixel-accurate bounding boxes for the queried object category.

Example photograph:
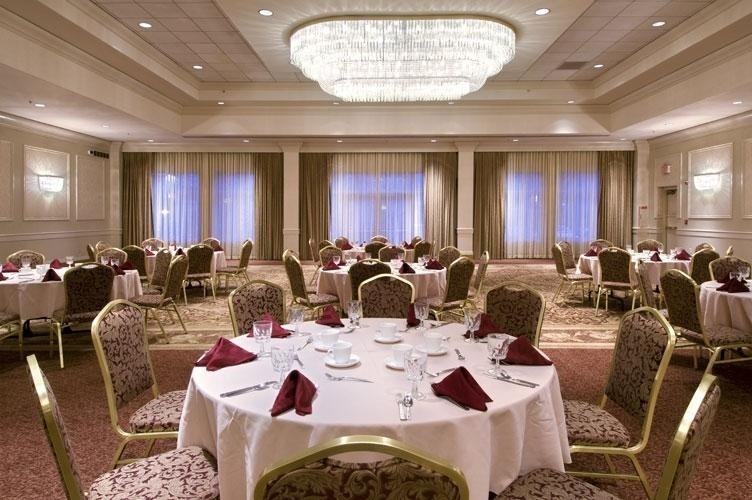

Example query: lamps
[288,15,518,104]
[36,174,64,193]
[692,171,721,191]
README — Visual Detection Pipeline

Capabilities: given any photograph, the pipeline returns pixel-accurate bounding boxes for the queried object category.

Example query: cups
[421,332,444,351]
[730,265,751,285]
[328,342,351,364]
[390,340,416,367]
[316,328,342,347]
[376,321,397,339]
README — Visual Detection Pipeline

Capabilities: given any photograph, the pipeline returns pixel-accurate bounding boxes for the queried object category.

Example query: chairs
[87,298,189,472]
[0,228,285,374]
[278,228,546,349]
[488,372,728,499]
[550,229,752,373]
[246,433,473,499]
[560,305,679,500]
[18,351,225,499]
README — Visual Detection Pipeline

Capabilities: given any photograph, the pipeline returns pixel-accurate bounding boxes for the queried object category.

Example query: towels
[431,365,495,414]
[265,369,321,418]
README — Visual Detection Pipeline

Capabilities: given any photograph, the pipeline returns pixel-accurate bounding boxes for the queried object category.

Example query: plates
[323,356,362,369]
[372,334,403,343]
[314,342,352,354]
[417,345,450,357]
[386,355,410,370]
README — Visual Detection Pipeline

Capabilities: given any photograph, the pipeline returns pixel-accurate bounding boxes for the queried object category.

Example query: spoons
[500,370,539,386]
[423,368,457,378]
[406,394,412,420]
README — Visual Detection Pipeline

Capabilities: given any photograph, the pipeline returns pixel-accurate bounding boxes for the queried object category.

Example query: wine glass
[485,331,512,378]
[403,355,425,403]
[254,320,273,357]
[413,301,429,334]
[621,241,681,261]
[15,255,121,273]
[461,309,483,348]
[269,341,299,387]
[288,303,306,336]
[330,250,432,270]
[346,299,362,329]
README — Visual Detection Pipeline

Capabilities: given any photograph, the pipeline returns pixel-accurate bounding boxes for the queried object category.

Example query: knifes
[396,391,405,423]
[492,376,534,388]
[219,379,275,398]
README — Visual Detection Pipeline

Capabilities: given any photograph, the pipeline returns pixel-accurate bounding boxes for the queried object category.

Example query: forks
[454,348,465,361]
[324,370,375,386]
